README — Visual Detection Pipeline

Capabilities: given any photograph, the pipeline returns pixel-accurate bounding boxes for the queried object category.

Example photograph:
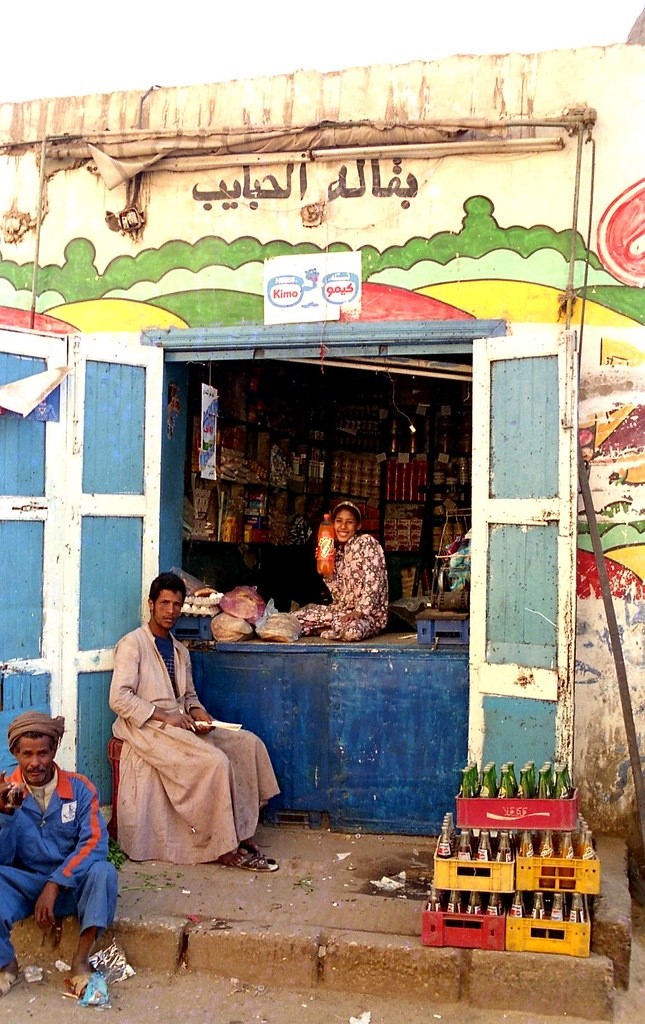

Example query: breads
[2,782,26,806]
[182,578,218,596]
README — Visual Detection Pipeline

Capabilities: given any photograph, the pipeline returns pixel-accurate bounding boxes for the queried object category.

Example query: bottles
[510,890,586,923]
[516,813,596,888]
[437,812,513,861]
[316,514,335,577]
[429,877,502,916]
[460,762,572,798]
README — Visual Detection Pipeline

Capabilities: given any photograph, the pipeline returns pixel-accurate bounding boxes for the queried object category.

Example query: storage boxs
[170,613,213,641]
[511,833,601,894]
[506,894,591,958]
[420,889,506,951]
[433,835,516,893]
[454,786,579,831]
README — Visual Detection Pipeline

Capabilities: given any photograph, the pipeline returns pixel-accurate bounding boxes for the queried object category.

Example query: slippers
[65,970,91,998]
[0,972,21,997]
[236,837,279,872]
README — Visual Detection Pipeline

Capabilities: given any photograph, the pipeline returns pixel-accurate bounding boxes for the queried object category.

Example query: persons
[292,499,388,642]
[109,572,282,872]
[0,711,118,1004]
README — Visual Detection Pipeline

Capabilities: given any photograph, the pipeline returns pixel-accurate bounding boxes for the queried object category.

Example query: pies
[211,591,303,641]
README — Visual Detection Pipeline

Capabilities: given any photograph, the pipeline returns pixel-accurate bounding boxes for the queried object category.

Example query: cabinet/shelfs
[183,366,329,612]
[326,368,471,633]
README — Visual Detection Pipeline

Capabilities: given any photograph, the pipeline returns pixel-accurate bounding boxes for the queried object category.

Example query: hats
[7,710,64,755]
